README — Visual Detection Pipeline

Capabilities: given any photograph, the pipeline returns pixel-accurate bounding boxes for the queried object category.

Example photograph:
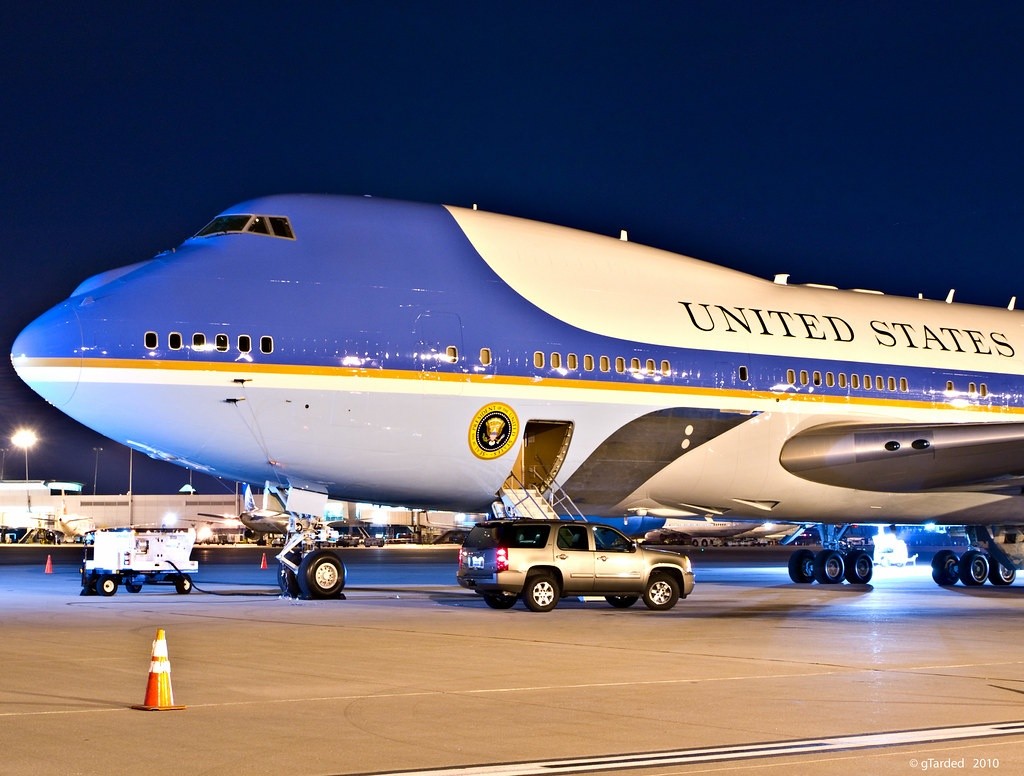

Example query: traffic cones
[259,553,269,570]
[44,554,53,573]
[131,629,187,712]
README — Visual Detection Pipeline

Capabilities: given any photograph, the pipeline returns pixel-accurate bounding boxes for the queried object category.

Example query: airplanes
[10,193,1024,599]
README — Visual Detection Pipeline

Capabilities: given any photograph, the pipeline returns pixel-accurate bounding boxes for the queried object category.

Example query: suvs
[457,519,696,611]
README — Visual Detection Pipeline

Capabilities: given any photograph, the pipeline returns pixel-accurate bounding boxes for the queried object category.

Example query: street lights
[93,447,103,495]
[0,448,9,480]
[11,429,38,512]
